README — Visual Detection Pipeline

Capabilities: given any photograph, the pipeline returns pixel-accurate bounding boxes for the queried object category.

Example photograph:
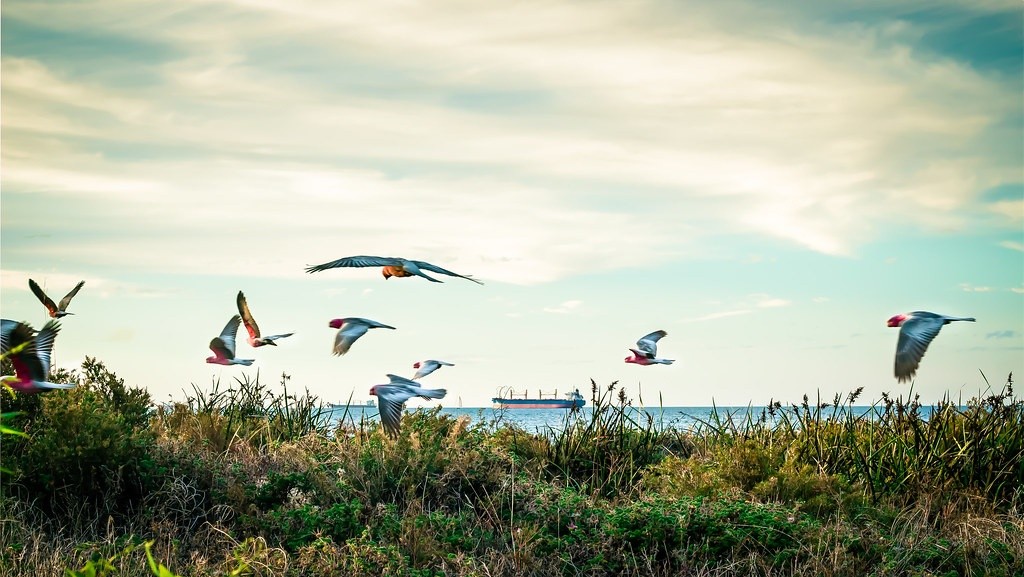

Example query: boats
[491,389,586,415]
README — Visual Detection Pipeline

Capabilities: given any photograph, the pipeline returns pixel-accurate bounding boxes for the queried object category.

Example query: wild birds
[206,314,254,369]
[27,278,86,319]
[887,309,978,385]
[237,290,295,347]
[412,359,455,383]
[1,318,76,403]
[623,329,675,366]
[328,316,397,357]
[308,254,484,284]
[369,374,448,441]
